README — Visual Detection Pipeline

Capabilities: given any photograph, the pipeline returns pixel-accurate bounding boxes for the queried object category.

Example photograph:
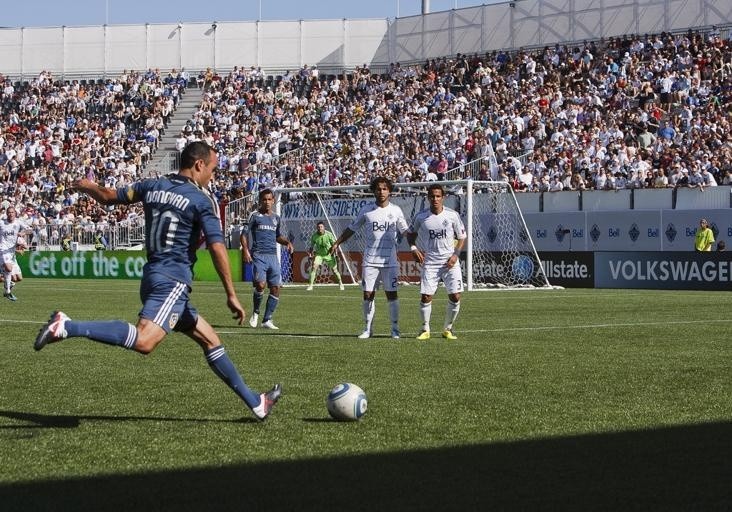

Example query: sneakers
[390,329,402,339]
[306,285,313,291]
[260,319,279,330]
[251,383,282,420]
[248,312,259,328]
[441,329,458,341]
[3,291,18,302]
[358,330,373,339]
[339,284,344,292]
[415,330,431,340]
[33,310,73,351]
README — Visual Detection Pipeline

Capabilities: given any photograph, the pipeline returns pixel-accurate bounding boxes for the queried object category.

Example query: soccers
[326,382,367,421]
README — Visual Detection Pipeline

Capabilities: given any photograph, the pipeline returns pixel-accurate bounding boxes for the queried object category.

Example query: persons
[305,222,344,291]
[33,141,285,420]
[328,176,411,339]
[0,207,27,301]
[92,230,107,251]
[0,69,186,228]
[241,189,294,331]
[59,231,74,251]
[694,219,715,252]
[715,239,725,251]
[176,28,732,191]
[404,184,466,339]
